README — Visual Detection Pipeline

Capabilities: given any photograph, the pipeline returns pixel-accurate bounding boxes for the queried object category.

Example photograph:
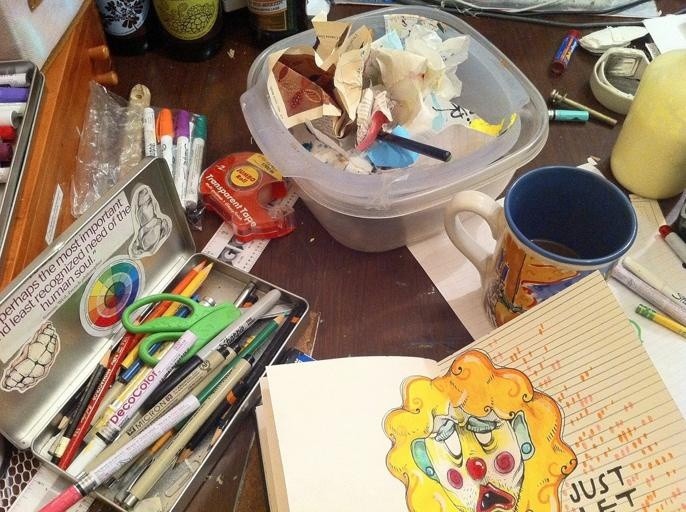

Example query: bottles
[610,48,685,200]
[93,0,306,64]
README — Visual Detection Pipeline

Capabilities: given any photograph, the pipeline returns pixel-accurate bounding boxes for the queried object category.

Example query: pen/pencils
[378,131,451,163]
[0,70,30,183]
[37,260,305,512]
[141,105,207,216]
[613,225,686,342]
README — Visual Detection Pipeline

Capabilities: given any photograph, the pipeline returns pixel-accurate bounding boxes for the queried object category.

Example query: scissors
[121,293,294,371]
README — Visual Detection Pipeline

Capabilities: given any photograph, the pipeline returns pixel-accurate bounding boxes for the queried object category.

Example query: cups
[443,166,639,327]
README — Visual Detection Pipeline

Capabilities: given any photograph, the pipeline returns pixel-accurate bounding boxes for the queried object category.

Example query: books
[254,271,686,512]
